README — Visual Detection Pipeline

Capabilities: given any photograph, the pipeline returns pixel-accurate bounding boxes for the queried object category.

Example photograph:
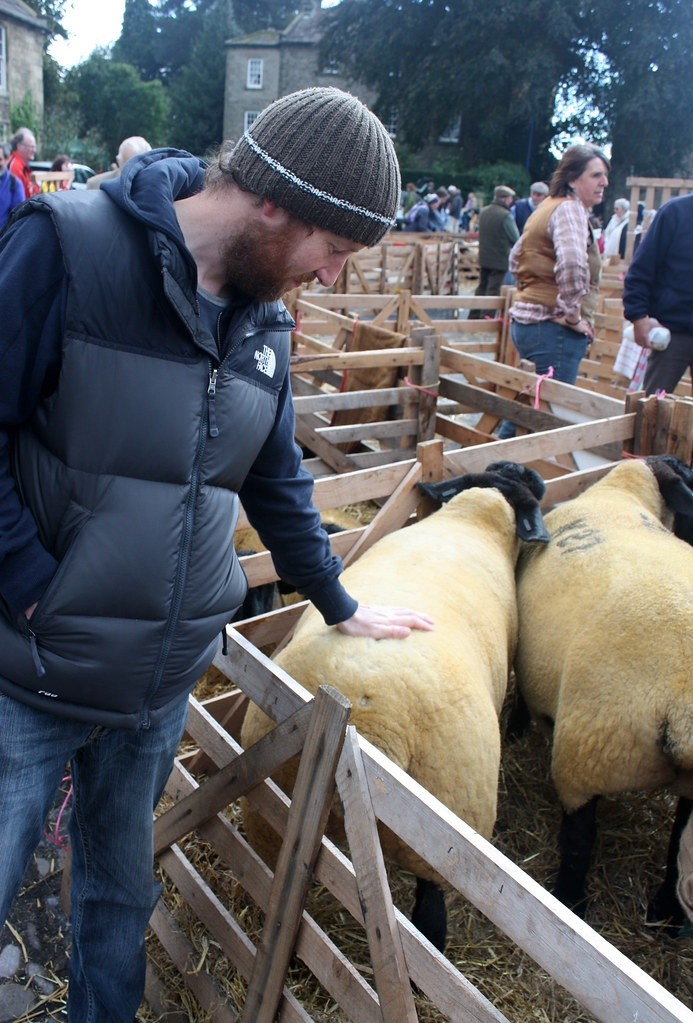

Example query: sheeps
[515,454,693,934]
[240,461,550,993]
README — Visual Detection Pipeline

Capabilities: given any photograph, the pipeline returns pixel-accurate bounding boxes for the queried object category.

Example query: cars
[29,159,97,190]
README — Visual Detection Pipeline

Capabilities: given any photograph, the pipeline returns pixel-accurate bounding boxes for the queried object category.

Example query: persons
[402,180,660,318]
[1,127,154,230]
[494,146,617,443]
[0,87,435,1023]
[622,196,693,397]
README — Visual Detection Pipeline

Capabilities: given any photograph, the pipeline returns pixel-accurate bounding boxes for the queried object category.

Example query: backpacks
[403,202,429,224]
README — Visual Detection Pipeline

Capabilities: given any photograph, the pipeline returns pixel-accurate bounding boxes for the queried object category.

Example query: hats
[495,186,515,199]
[229,88,402,249]
[531,182,549,196]
